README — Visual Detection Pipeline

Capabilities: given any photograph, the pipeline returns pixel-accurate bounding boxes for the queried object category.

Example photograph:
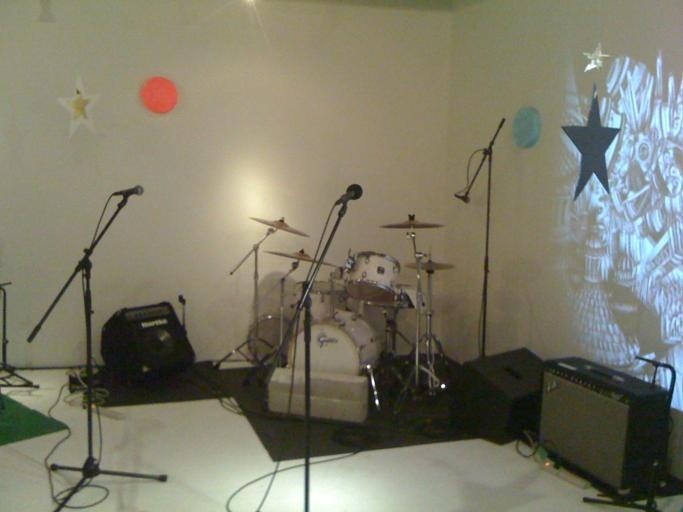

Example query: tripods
[214,227,302,385]
[50,259,168,512]
[375,225,449,397]
[582,354,675,511]
[0,283,36,387]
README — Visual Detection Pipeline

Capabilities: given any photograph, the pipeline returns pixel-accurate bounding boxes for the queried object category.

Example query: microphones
[335,184,363,205]
[113,185,143,196]
[453,193,470,202]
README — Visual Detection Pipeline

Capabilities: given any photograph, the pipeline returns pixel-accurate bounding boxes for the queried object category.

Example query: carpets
[218,352,495,462]
[0,392,67,448]
[95,360,231,406]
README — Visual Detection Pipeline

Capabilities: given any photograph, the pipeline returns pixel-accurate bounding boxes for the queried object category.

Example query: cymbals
[249,216,309,237]
[264,250,336,267]
[407,261,454,270]
[380,221,446,228]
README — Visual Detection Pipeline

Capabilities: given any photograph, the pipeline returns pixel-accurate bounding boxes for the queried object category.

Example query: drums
[293,280,348,335]
[364,284,414,308]
[286,310,378,387]
[248,314,291,367]
[346,251,400,302]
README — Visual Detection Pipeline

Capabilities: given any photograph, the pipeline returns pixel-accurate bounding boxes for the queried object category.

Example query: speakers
[446,348,542,438]
[102,301,195,387]
[538,357,668,492]
